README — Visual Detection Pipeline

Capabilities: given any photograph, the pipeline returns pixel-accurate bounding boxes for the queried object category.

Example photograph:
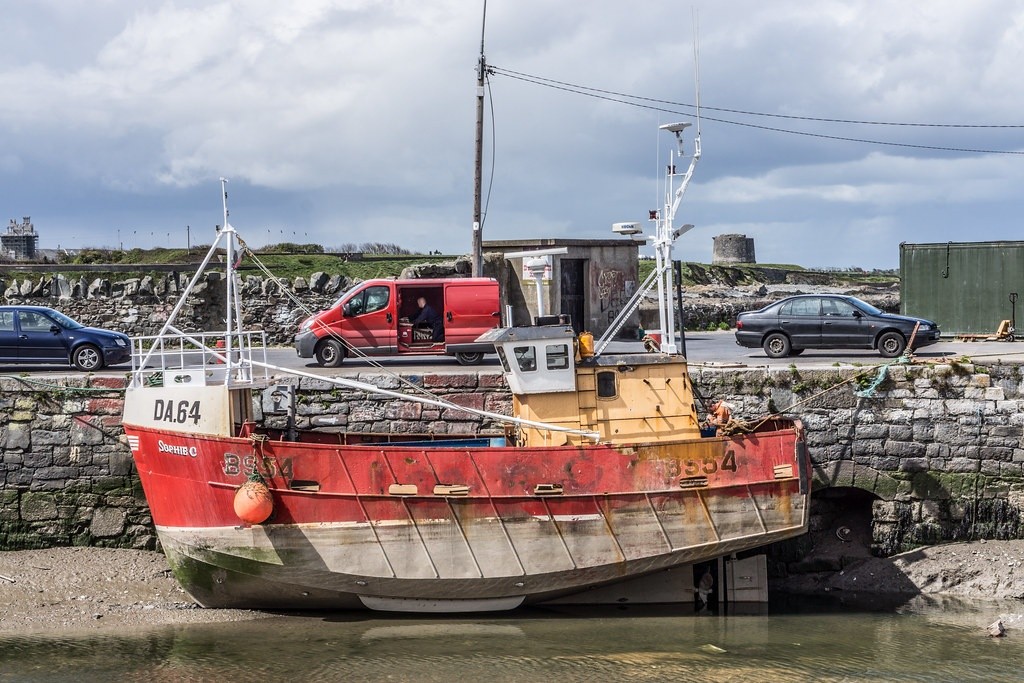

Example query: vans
[294,278,501,367]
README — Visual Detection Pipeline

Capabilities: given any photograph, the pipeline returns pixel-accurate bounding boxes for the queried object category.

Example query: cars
[0,305,131,372]
[735,294,940,358]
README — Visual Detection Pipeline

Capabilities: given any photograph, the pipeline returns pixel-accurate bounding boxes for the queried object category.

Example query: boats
[121,1,810,616]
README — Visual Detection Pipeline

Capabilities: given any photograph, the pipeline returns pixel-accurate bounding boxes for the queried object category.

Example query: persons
[412,297,445,343]
